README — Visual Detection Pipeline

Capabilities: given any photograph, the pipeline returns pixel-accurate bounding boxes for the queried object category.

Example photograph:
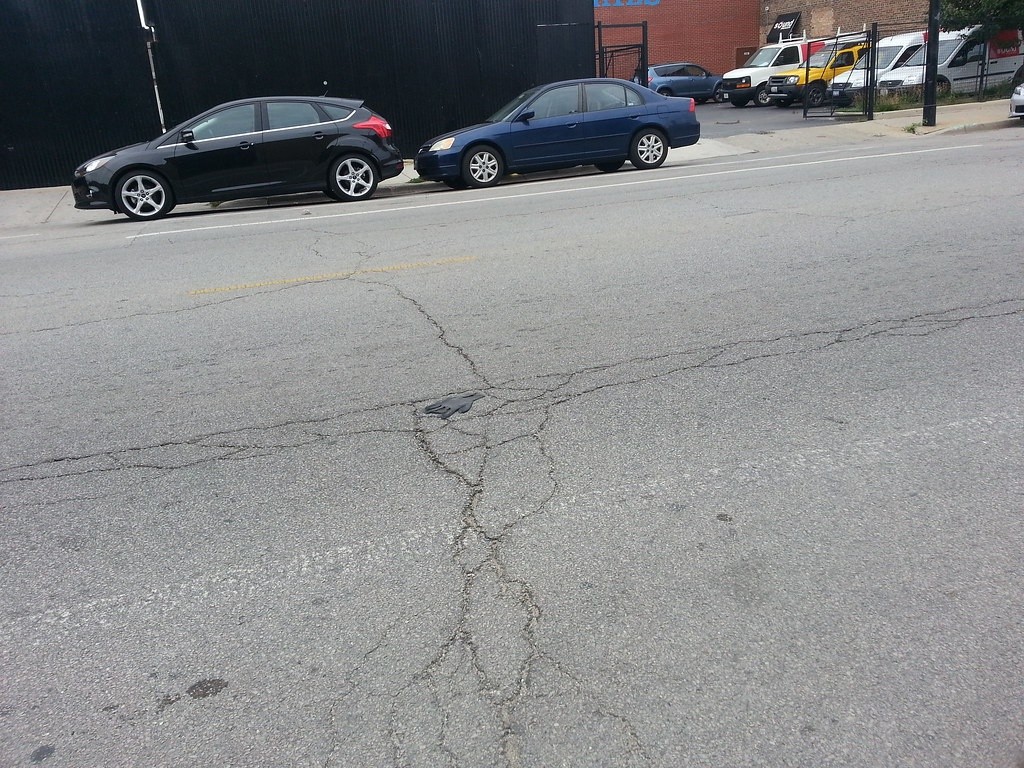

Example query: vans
[878,23,1024,103]
[825,29,946,107]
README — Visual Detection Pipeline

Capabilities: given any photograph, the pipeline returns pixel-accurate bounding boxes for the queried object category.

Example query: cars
[1009,82,1024,120]
[416,78,700,186]
[72,98,402,219]
[628,62,728,102]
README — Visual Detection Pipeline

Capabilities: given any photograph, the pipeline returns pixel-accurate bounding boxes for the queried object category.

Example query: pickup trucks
[718,28,867,108]
[765,30,875,107]
